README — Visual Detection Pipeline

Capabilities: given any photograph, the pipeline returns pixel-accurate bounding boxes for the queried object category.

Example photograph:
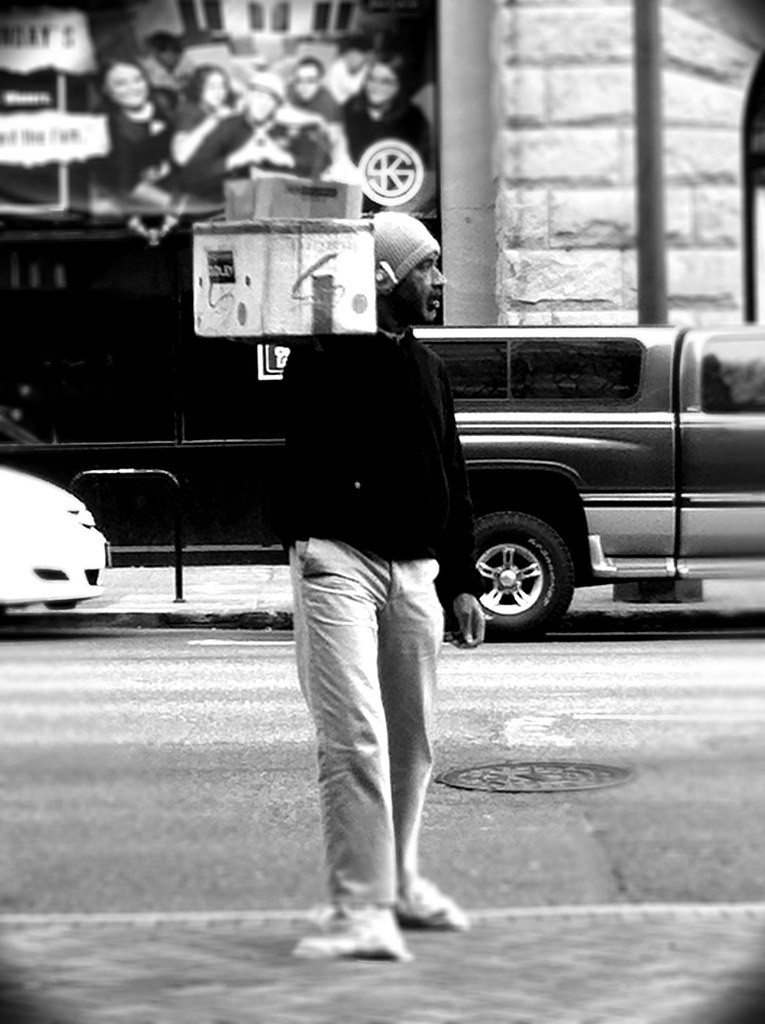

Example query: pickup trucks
[411,323,765,646]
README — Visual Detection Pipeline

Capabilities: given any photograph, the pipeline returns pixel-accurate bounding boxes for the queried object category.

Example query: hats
[371,210,441,294]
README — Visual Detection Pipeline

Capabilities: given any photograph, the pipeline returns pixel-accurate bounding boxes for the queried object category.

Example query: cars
[0,462,114,617]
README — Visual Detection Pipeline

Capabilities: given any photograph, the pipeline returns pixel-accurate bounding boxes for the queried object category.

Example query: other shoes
[396,879,469,933]
[332,907,401,959]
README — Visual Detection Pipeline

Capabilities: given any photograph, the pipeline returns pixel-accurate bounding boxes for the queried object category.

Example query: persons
[97,26,438,215]
[272,205,489,957]
